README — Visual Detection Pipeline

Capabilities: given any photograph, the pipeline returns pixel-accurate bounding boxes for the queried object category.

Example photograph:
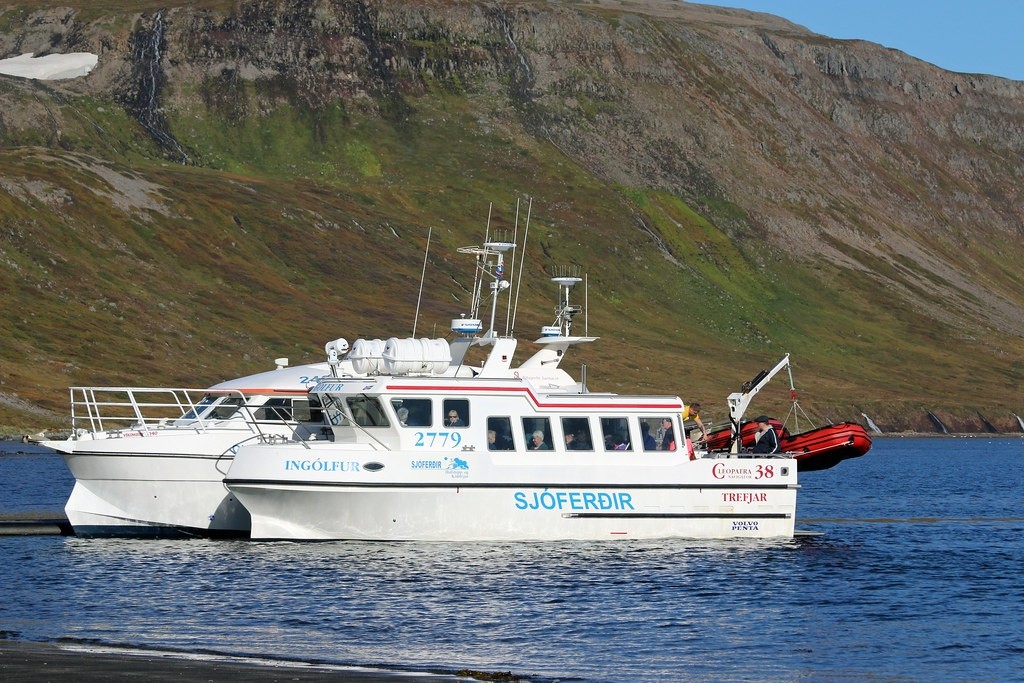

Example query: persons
[397,407,409,426]
[611,434,628,450]
[662,403,707,450]
[641,422,656,450]
[565,427,582,450]
[488,430,497,450]
[447,410,465,426]
[753,416,781,457]
[530,430,548,450]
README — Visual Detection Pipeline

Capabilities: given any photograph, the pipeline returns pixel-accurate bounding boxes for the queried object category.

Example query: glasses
[449,416,457,418]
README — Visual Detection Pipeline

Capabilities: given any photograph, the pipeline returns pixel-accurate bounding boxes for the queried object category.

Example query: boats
[27,247,620,541]
[224,270,824,543]
[696,417,873,469]
[23,434,50,446]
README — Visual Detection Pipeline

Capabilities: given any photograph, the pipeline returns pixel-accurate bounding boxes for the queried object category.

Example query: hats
[754,416,768,422]
